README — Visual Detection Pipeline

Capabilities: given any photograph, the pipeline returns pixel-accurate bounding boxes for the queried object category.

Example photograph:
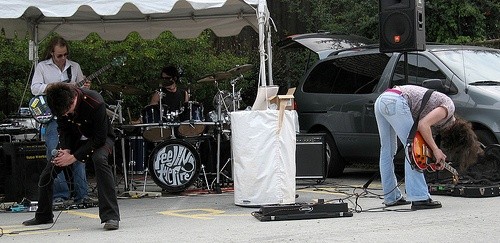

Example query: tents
[0,0,277,111]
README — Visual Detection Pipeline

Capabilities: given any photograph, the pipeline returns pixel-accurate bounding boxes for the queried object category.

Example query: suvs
[276,32,500,179]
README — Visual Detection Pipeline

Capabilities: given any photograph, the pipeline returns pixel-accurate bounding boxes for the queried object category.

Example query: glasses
[54,52,68,59]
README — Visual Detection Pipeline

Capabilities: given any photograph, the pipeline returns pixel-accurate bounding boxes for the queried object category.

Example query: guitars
[28,55,128,124]
[405,131,460,177]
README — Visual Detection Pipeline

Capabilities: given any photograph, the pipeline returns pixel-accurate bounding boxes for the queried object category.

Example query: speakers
[296,133,327,179]
[17,146,71,201]
[378,0,425,52]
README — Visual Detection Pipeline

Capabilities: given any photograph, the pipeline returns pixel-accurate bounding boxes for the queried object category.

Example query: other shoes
[74,197,99,206]
[53,199,63,205]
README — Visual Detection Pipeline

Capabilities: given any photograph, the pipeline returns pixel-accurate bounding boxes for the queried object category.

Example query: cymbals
[227,64,254,77]
[196,71,232,82]
[101,83,145,96]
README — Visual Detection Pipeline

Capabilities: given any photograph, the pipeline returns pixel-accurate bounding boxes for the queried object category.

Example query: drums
[146,139,202,194]
[141,104,172,142]
[205,109,230,142]
[175,100,206,138]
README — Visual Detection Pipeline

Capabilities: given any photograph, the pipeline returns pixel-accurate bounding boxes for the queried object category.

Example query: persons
[151,65,189,120]
[374,85,483,210]
[22,82,120,230]
[31,36,98,207]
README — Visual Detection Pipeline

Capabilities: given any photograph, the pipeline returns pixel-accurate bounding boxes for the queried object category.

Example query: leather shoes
[104,220,119,229]
[411,198,442,211]
[385,196,411,207]
[22,218,53,226]
